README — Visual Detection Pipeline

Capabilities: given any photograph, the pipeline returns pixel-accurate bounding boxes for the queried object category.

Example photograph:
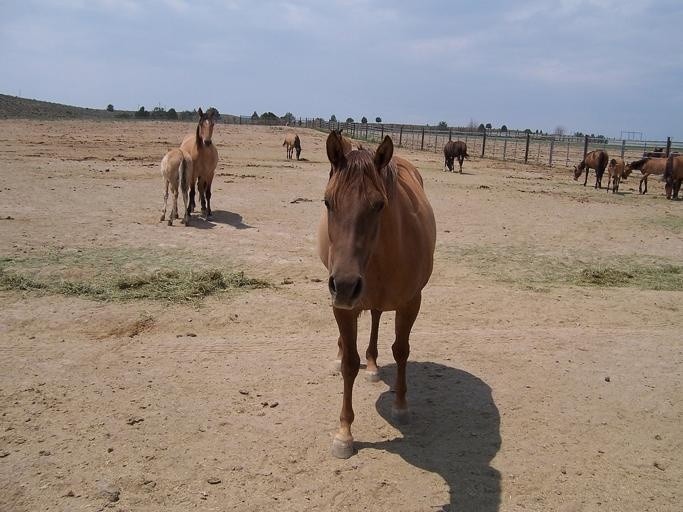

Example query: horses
[319,129,436,458]
[160,148,193,226]
[180,108,218,222]
[282,133,303,160]
[443,141,470,173]
[606,148,683,200]
[573,150,609,190]
